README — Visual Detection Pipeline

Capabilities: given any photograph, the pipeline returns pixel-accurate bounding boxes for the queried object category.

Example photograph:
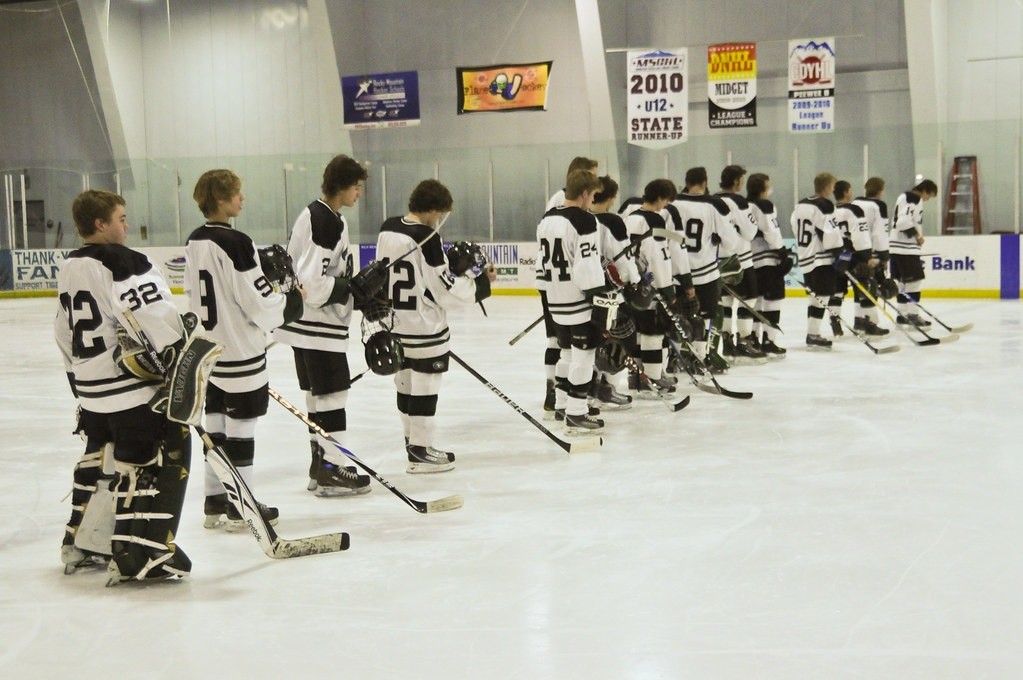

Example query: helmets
[365,330,405,376]
[595,338,628,375]
[449,239,486,278]
[623,282,656,312]
[878,278,901,299]
[257,243,295,280]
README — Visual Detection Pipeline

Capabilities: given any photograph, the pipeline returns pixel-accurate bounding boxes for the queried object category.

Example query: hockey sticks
[445,349,606,454]
[788,271,900,355]
[668,338,720,396]
[653,289,753,399]
[508,228,686,346]
[720,283,784,335]
[266,388,465,514]
[845,271,940,348]
[614,335,691,413]
[901,291,974,333]
[121,305,350,560]
[877,294,960,344]
[383,208,453,270]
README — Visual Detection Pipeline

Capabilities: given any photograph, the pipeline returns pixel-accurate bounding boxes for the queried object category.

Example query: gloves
[776,246,793,275]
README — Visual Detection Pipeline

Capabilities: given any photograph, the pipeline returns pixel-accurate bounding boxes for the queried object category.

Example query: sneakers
[314,445,371,498]
[405,445,456,473]
[203,493,231,529]
[225,500,280,532]
[831,317,843,342]
[666,331,785,376]
[806,334,832,352]
[545,365,677,430]
[854,315,932,340]
[307,440,358,491]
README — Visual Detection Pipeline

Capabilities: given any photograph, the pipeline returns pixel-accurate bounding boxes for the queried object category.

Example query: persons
[889,180,938,332]
[363,179,498,475]
[271,155,390,497]
[53,189,220,589]
[184,169,307,534]
[533,155,794,438]
[789,172,892,353]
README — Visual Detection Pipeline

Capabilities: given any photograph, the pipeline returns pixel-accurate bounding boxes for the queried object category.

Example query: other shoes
[105,547,175,586]
[60,534,107,576]
